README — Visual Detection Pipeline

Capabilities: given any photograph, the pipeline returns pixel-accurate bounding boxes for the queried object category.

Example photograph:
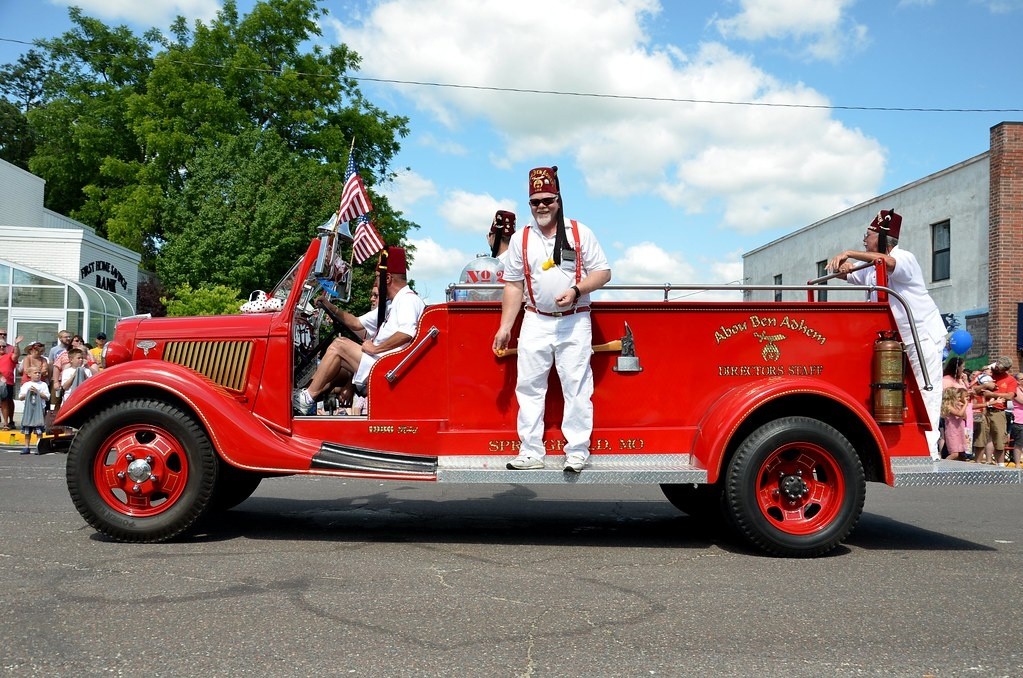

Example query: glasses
[0,345,6,349]
[529,196,557,206]
[369,293,379,299]
[0,333,7,336]
[489,232,495,237]
[864,232,877,238]
[73,339,82,343]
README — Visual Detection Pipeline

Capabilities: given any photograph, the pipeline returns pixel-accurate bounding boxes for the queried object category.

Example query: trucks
[35,228,1022,560]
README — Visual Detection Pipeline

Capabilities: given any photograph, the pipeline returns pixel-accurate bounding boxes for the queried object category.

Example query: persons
[0,329,24,430]
[938,356,1023,469]
[493,168,613,473]
[488,210,516,263]
[824,209,948,462]
[293,248,424,416]
[18,330,107,454]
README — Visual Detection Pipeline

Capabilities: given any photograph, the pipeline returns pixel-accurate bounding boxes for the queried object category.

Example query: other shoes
[10,423,17,429]
[20,448,30,454]
[33,448,38,455]
[2,424,12,431]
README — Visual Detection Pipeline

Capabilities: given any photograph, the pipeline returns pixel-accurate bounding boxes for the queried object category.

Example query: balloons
[942,348,948,361]
[950,330,973,355]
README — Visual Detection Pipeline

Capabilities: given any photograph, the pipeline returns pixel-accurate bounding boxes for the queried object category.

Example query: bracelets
[15,345,18,347]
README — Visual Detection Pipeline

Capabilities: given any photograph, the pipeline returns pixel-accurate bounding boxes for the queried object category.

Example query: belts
[526,306,590,316]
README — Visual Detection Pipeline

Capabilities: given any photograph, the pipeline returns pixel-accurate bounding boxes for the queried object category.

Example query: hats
[23,341,37,352]
[996,355,1013,371]
[95,332,107,339]
[491,210,516,236]
[376,246,406,274]
[528,166,560,197]
[868,209,903,239]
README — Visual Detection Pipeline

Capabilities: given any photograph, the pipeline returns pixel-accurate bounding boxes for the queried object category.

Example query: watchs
[572,286,582,301]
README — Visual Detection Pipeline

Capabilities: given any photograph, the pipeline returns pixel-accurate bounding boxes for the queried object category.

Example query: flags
[353,215,386,264]
[336,156,373,225]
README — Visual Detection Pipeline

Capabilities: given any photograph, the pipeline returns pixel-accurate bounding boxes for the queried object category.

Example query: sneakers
[293,388,313,416]
[505,455,544,470]
[562,457,585,473]
